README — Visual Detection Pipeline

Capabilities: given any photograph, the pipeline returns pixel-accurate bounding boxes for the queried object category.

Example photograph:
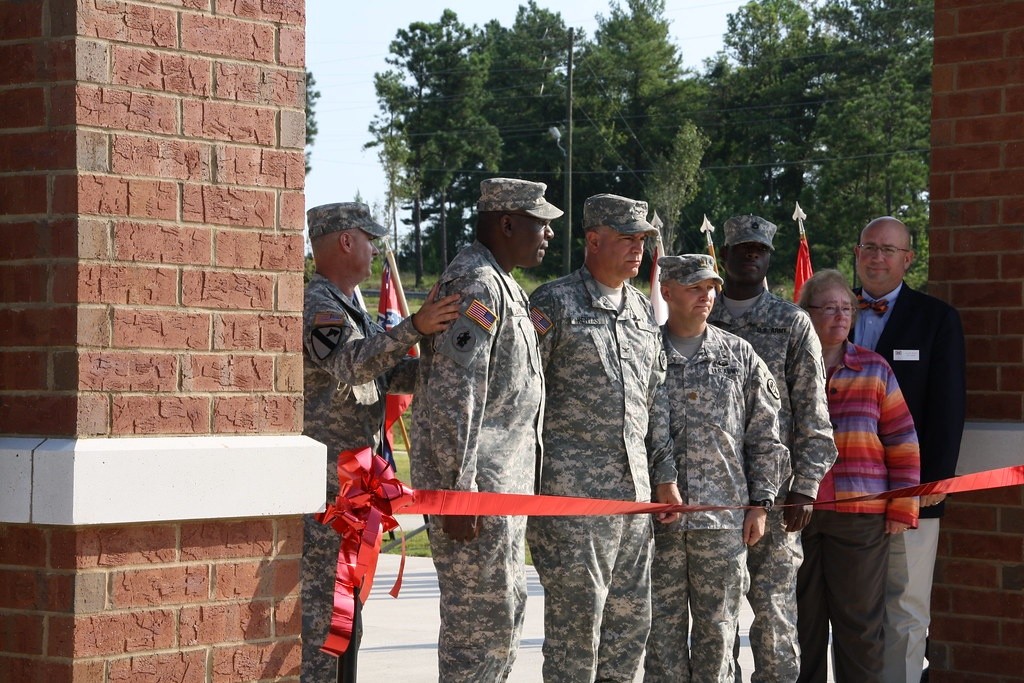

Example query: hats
[583,193,660,237]
[658,253,724,286]
[307,201,388,239]
[477,178,564,220]
[724,215,777,251]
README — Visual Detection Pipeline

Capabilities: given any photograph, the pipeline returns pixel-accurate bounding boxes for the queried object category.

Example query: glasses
[858,244,911,256]
[804,306,856,315]
[502,212,551,230]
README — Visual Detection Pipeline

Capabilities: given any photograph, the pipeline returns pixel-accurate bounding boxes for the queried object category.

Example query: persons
[301,203,462,683]
[848,217,967,683]
[644,255,792,683]
[527,194,684,683]
[797,271,920,683]
[706,216,840,683]
[411,177,564,683]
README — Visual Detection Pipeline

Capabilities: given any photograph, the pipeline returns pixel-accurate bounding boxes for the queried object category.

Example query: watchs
[750,500,772,512]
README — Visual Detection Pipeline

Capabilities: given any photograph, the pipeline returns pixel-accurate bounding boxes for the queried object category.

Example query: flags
[650,247,669,326]
[794,240,813,304]
[376,256,419,450]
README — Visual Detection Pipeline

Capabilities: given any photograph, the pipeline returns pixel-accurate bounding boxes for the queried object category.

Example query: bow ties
[858,295,889,318]
